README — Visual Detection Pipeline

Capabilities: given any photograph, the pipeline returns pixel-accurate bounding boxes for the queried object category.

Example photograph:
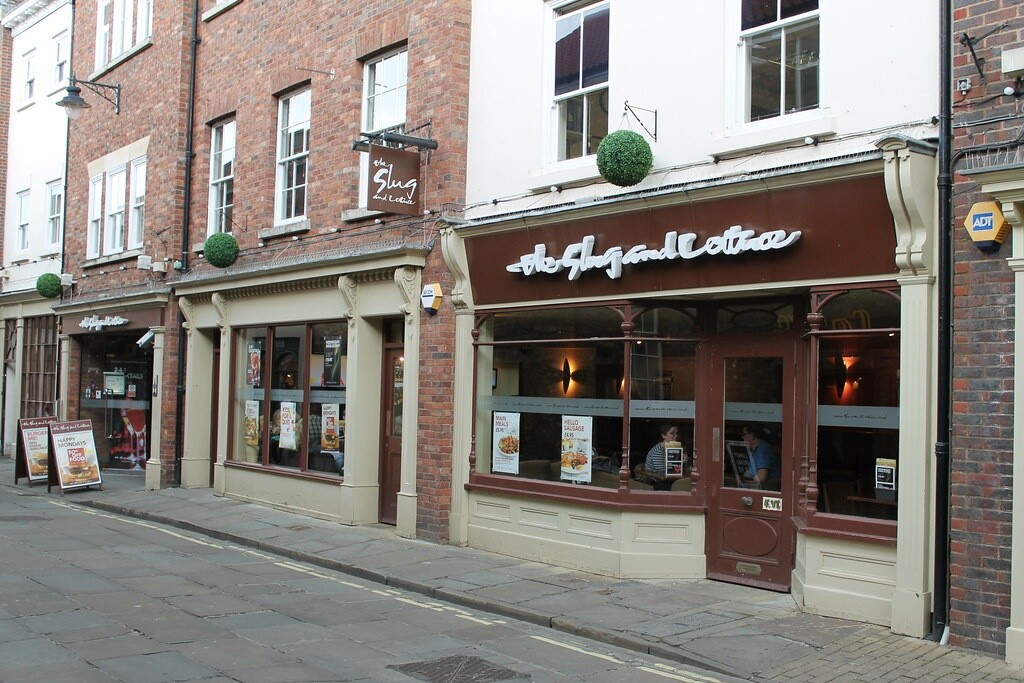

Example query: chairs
[520,461,552,480]
[857,479,875,512]
[635,463,671,490]
[847,495,897,520]
[823,481,856,515]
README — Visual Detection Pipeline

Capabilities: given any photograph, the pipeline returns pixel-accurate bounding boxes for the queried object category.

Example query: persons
[742,425,776,489]
[645,424,689,490]
[272,404,321,452]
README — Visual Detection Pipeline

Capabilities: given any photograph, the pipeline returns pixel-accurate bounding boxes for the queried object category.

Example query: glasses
[667,432,679,435]
[741,433,749,437]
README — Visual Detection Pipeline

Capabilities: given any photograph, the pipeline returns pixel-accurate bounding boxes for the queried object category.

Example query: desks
[636,470,731,481]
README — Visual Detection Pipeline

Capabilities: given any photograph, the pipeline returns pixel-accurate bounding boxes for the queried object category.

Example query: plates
[497,436,518,457]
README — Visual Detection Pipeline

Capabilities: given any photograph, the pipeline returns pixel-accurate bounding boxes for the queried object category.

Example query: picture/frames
[492,368,498,389]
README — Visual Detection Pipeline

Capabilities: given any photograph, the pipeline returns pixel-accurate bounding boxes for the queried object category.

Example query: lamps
[55,71,121,121]
